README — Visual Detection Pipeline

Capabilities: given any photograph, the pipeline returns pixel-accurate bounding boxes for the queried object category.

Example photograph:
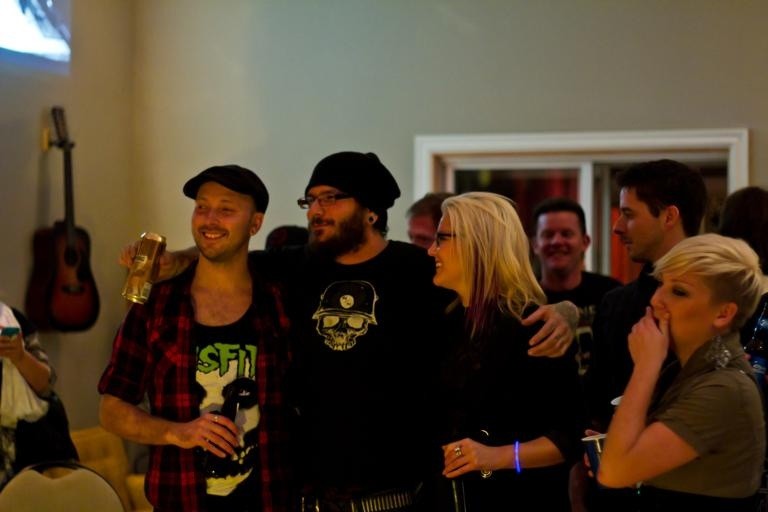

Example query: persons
[95,151,768,512]
[0,300,57,492]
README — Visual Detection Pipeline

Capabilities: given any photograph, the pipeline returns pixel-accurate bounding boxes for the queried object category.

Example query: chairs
[0,456,126,512]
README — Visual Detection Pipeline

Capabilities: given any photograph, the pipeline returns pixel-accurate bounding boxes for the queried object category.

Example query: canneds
[581,434,605,494]
[120,230,167,307]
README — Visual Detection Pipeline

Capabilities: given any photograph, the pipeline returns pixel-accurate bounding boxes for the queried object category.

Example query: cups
[581,432,608,478]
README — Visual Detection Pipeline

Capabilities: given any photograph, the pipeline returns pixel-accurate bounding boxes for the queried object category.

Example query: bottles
[197,390,239,478]
[744,302,767,388]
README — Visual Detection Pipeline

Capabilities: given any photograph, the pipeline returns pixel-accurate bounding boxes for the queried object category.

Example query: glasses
[297,193,356,205]
[435,232,456,249]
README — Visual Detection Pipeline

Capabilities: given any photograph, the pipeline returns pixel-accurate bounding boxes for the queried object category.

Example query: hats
[310,279,376,320]
[182,166,269,212]
[304,153,401,209]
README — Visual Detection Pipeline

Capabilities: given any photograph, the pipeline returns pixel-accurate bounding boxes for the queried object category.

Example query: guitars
[24,107,100,331]
